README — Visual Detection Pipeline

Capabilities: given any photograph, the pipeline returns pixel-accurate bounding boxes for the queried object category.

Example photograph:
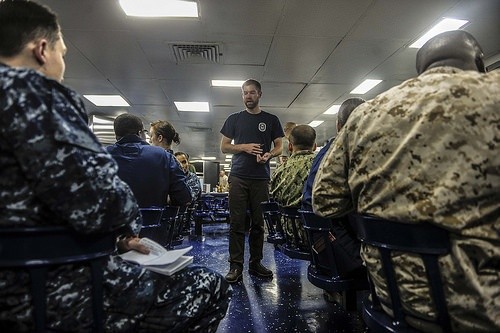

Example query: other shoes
[248,260,273,278]
[225,264,244,282]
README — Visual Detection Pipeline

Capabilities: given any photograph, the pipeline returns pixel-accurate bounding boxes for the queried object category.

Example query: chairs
[276,205,312,261]
[259,201,287,245]
[0,226,117,333]
[297,208,371,313]
[138,193,252,252]
[350,214,500,333]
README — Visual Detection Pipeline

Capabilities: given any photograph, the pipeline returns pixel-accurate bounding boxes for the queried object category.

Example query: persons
[0,0,233,333]
[270,30,500,333]
[220,80,286,282]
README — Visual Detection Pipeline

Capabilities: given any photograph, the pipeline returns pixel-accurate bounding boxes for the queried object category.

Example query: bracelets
[269,152,273,159]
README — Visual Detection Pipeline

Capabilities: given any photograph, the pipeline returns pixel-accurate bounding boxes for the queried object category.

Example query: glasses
[142,129,148,133]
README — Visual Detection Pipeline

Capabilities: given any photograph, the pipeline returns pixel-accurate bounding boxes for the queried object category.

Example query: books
[118,238,194,276]
